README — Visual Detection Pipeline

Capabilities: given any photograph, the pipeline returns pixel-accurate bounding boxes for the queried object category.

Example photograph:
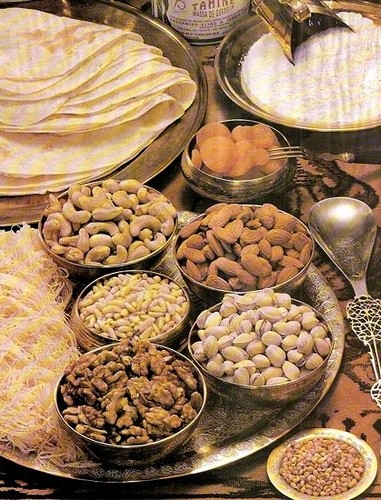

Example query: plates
[1,214,345,485]
[0,0,210,234]
[267,427,379,500]
[211,0,381,143]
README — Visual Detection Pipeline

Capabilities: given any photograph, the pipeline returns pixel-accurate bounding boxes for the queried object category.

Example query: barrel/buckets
[149,0,252,46]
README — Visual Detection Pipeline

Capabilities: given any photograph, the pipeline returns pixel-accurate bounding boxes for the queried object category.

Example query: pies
[0,7,198,197]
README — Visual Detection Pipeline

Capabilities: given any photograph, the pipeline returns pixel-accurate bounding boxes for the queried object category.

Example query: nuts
[176,203,313,291]
[191,287,332,388]
[61,336,203,445]
[77,273,189,342]
[44,178,177,266]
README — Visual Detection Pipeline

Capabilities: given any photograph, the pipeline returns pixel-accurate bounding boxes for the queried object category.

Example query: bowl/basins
[36,112,338,470]
